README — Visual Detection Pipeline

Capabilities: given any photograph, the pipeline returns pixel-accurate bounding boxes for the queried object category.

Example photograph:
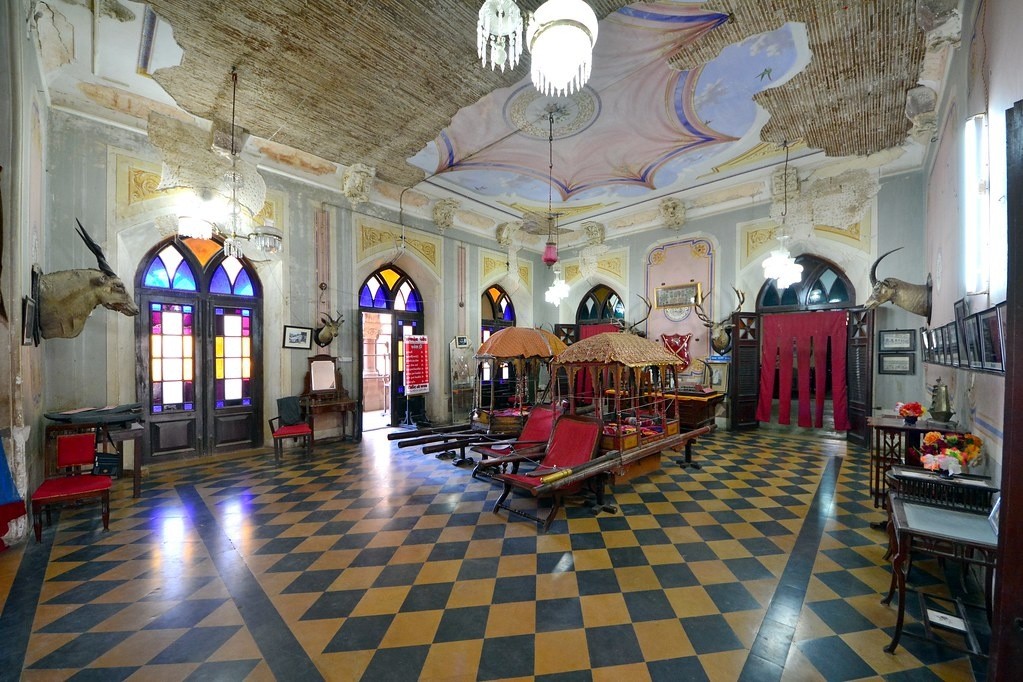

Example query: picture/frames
[653,281,704,309]
[878,351,915,375]
[704,361,730,395]
[283,325,314,351]
[918,296,1007,377]
[879,329,917,352]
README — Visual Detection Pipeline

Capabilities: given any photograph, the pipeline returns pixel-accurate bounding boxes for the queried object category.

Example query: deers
[607,293,652,337]
[694,285,746,349]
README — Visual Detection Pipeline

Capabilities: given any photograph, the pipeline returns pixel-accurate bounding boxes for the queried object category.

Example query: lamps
[542,111,559,272]
[762,235,805,290]
[521,1,601,99]
[475,2,525,70]
[175,68,284,261]
[543,270,572,308]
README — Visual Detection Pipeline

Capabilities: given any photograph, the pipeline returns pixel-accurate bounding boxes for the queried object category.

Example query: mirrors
[306,353,339,394]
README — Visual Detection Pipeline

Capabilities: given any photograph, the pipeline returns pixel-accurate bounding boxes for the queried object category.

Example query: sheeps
[318,311,345,346]
[38,217,139,340]
[864,246,928,317]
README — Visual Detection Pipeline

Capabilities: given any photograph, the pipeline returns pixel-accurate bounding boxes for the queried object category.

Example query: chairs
[492,415,609,533]
[472,403,565,487]
[267,396,317,461]
[30,421,112,540]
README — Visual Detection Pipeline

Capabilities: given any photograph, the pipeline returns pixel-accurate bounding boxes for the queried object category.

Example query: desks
[70,420,145,497]
[879,495,1000,657]
[864,406,998,584]
[298,394,362,448]
